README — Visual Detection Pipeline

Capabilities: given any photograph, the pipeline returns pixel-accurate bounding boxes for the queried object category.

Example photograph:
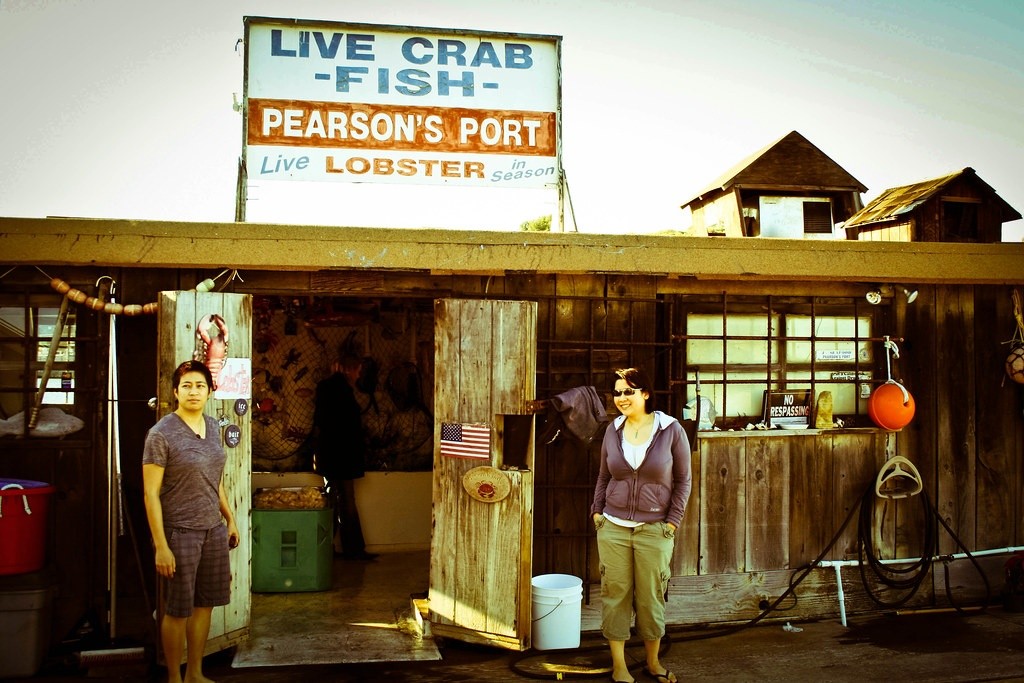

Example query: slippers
[645,667,677,683]
[611,675,638,683]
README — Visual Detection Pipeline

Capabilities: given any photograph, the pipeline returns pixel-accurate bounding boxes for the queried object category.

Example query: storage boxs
[250,486,331,594]
[0,583,50,677]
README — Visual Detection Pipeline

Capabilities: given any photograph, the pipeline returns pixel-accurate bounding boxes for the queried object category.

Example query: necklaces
[628,416,648,438]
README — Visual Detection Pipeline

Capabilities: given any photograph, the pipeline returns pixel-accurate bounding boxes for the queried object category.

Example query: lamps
[865,284,889,305]
[896,284,919,303]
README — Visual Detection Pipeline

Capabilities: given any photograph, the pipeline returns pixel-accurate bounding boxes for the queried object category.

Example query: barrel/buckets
[0,477,56,573]
[528,574,583,648]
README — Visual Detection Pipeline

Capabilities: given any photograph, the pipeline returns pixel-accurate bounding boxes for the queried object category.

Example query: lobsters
[191,312,229,391]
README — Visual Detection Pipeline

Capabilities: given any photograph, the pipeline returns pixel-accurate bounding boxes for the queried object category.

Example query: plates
[780,424,809,430]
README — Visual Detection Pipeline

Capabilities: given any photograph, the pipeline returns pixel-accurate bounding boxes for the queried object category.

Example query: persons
[314,351,379,559]
[143,361,239,683]
[590,368,693,683]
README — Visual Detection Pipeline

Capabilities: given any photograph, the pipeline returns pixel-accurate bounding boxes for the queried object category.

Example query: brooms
[80,436,144,669]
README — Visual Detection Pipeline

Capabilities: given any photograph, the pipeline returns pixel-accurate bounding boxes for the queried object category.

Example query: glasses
[613,388,642,397]
